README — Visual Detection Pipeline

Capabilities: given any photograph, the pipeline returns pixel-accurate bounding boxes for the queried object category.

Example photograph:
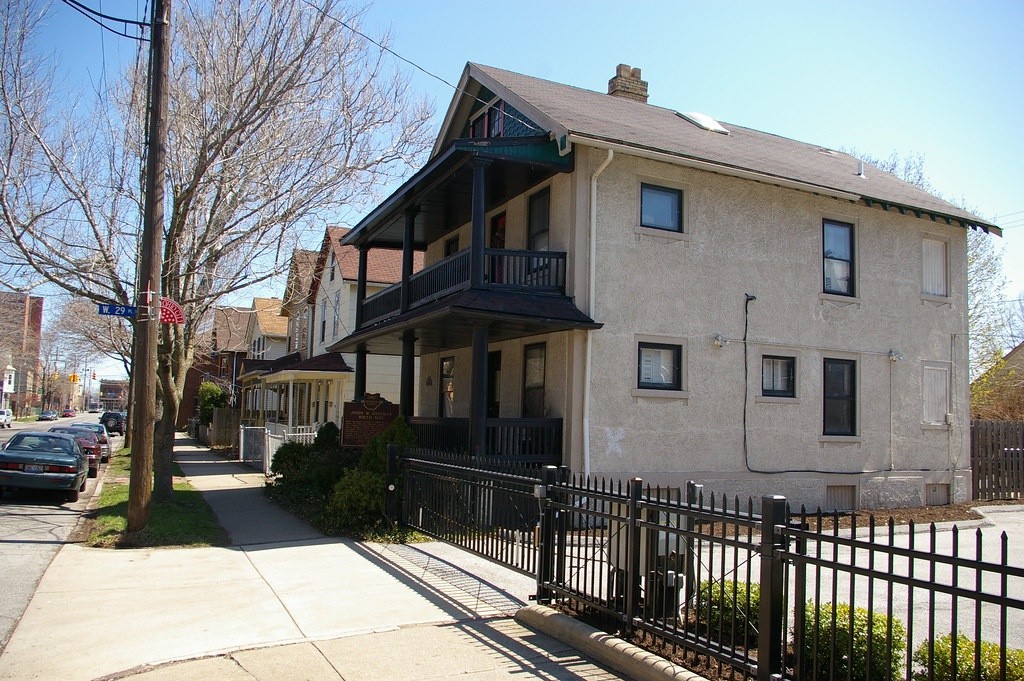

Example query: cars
[39,409,59,420]
[61,423,112,463]
[37,426,108,478]
[62,409,76,417]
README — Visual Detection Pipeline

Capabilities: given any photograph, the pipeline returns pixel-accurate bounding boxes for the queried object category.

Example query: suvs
[0,430,94,503]
[98,411,124,436]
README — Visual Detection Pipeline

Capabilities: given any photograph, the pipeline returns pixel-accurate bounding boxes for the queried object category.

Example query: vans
[0,408,13,429]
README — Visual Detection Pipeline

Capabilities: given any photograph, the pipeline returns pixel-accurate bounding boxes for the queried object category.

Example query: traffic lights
[92,372,96,380]
[76,375,79,383]
[70,375,75,383]
[54,373,58,380]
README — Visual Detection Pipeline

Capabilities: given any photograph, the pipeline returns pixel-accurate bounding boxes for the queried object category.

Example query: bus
[89,401,104,413]
